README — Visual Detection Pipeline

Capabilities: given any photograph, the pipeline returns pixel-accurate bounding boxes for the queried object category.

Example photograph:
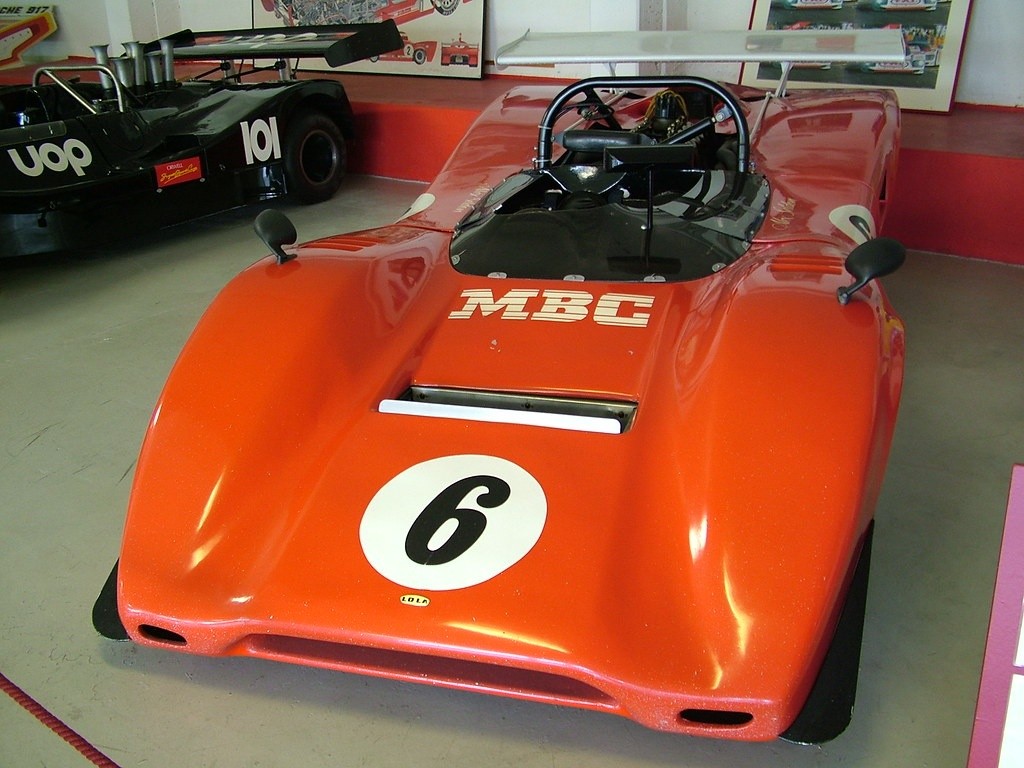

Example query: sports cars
[0,19,406,276]
[91,29,907,746]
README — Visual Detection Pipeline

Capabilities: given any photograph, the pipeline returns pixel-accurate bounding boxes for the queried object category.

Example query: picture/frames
[738,0,975,116]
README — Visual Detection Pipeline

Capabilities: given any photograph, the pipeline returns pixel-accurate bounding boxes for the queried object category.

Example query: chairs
[544,131,641,197]
